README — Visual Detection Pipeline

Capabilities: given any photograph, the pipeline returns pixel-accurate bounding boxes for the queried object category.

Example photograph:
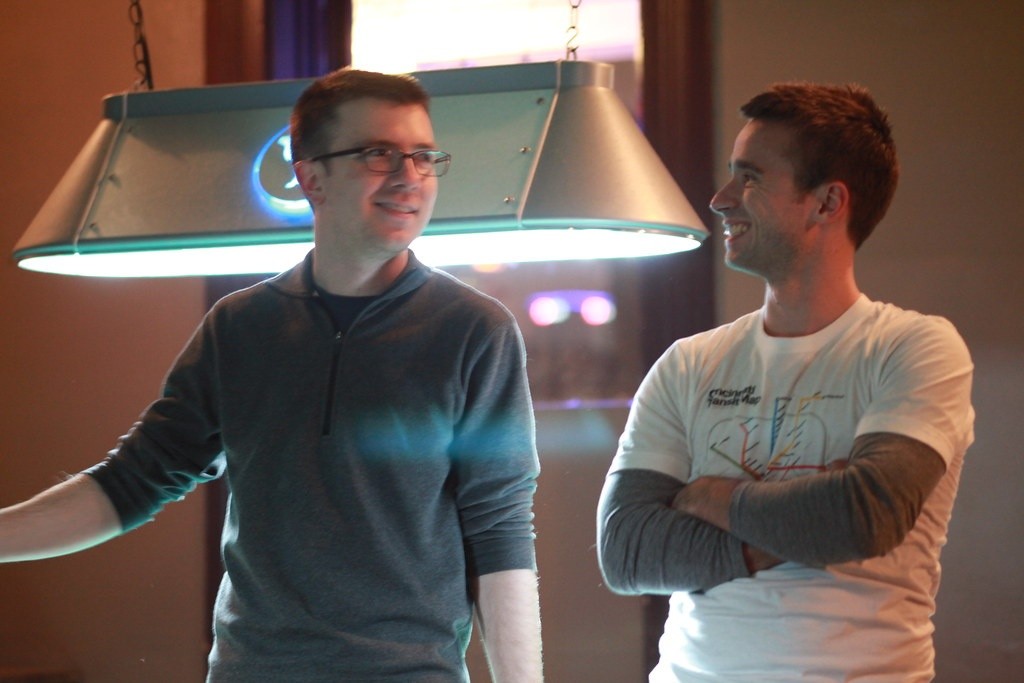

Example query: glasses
[309,144,453,177]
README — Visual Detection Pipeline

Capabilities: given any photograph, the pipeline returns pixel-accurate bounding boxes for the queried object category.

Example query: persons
[595,83,975,682]
[0,68,544,683]
